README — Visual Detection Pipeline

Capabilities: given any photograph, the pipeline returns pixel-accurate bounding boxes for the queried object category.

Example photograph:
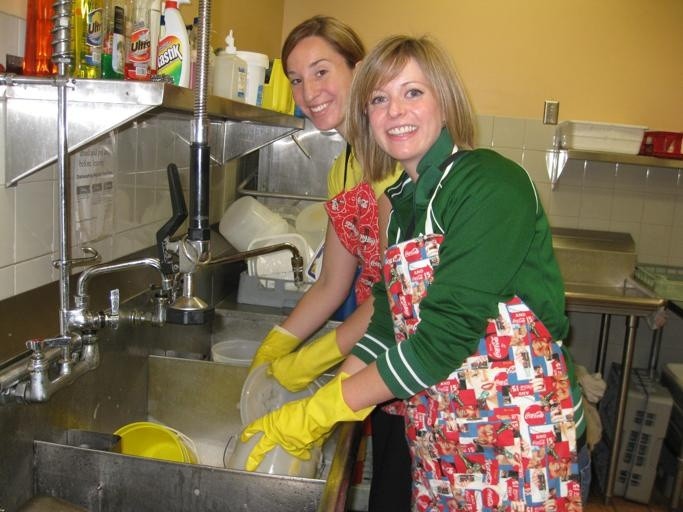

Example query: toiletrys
[24,0,152,80]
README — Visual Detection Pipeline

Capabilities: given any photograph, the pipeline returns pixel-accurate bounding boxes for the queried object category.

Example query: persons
[237,33,608,510]
[244,14,404,394]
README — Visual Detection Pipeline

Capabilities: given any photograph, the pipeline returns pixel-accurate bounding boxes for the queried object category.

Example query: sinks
[95,313,361,377]
[0,439,352,512]
[0,355,362,439]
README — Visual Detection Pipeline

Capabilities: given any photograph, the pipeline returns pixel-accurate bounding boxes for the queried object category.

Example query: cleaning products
[213,30,247,102]
[157,0,192,89]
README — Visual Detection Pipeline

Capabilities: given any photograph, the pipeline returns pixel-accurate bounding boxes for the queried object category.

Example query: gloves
[240,326,376,475]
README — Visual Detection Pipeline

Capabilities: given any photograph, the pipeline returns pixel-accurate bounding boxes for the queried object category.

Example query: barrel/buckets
[217,51,269,106]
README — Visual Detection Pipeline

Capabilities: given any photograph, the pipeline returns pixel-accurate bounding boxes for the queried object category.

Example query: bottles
[189,16,199,89]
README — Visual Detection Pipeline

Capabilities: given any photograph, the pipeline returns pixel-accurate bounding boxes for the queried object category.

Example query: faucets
[77,257,172,294]
[205,243,303,283]
